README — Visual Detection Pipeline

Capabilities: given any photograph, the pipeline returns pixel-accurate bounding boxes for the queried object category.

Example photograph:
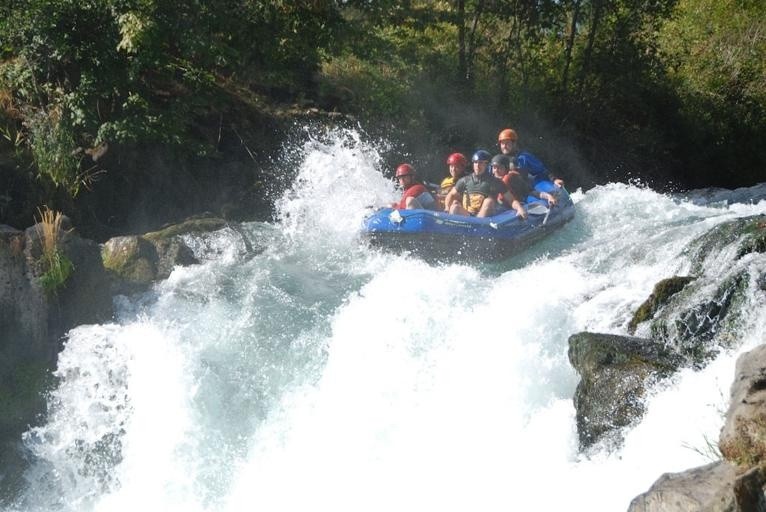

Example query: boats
[366,178,578,265]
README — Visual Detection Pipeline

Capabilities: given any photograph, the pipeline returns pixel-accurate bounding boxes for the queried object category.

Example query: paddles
[489,203,547,230]
[540,189,561,229]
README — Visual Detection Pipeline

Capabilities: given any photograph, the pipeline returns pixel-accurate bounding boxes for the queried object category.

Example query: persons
[390,163,443,212]
[497,128,565,189]
[488,155,558,207]
[437,152,470,209]
[442,149,528,223]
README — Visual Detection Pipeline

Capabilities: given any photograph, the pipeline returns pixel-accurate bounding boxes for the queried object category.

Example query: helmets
[491,154,510,167]
[446,152,468,167]
[498,128,518,142]
[472,150,492,163]
[395,163,417,177]
